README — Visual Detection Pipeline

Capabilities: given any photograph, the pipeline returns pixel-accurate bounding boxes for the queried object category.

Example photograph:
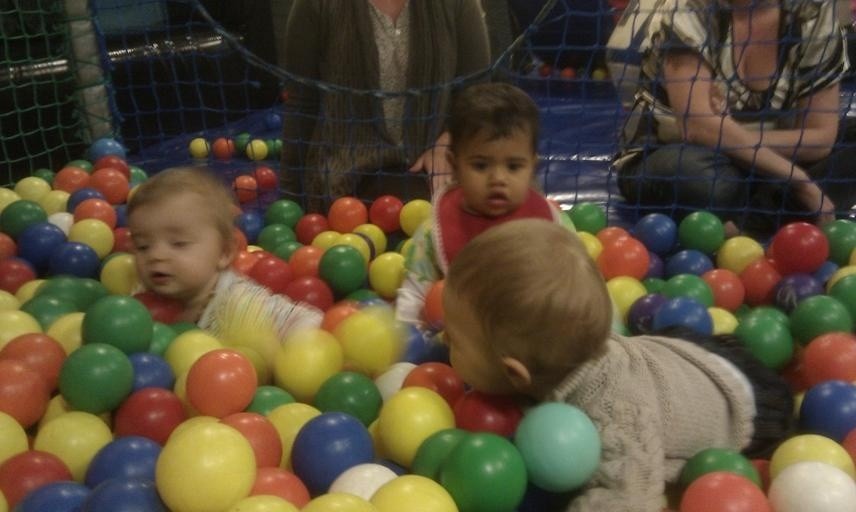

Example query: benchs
[533,95,625,209]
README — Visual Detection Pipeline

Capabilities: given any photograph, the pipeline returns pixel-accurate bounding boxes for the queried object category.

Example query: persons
[279,0,491,211]
[607,0,855,236]
[125,166,326,346]
[387,83,792,512]
